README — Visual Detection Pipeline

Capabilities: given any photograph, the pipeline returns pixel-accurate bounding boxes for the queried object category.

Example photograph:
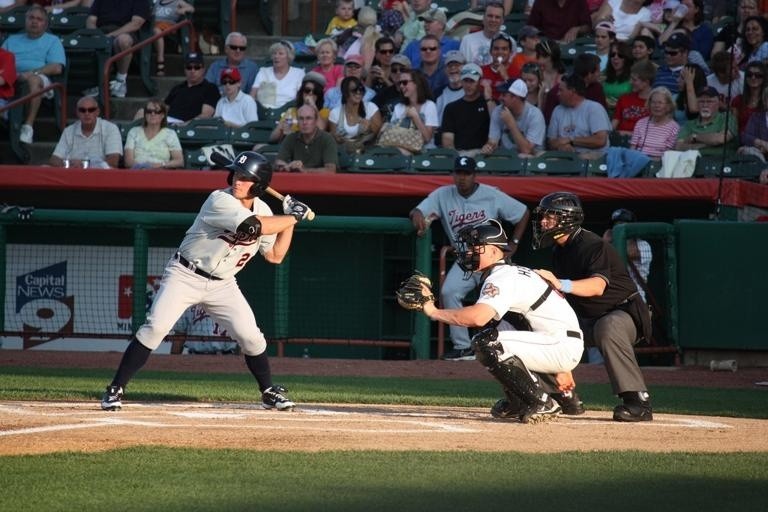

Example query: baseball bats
[210,152,315,220]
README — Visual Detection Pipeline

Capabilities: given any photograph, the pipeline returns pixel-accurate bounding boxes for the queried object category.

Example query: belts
[174,252,223,281]
[567,330,580,339]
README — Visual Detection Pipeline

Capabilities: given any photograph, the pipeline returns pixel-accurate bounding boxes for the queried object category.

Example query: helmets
[454,219,512,280]
[224,150,273,197]
[532,192,584,252]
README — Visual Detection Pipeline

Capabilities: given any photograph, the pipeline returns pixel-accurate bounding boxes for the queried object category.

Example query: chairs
[0,0,768,180]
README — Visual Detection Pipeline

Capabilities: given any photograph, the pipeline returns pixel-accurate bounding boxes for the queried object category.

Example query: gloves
[290,202,312,221]
[283,194,299,215]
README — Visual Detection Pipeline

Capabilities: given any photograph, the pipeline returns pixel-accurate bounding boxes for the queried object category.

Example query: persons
[101,150,310,412]
[529,190,653,420]
[603,208,652,304]
[397,222,583,425]
[0,0,767,177]
[409,158,530,362]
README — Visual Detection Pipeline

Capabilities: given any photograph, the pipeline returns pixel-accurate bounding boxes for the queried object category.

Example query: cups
[709,360,738,372]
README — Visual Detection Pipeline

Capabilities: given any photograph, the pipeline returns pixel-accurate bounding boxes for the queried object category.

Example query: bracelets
[557,278,571,294]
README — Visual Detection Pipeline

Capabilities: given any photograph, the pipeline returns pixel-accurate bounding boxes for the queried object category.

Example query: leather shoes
[552,389,587,415]
[612,398,652,421]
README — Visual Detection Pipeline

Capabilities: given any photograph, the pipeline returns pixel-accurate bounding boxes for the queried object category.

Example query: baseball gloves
[398,271,432,309]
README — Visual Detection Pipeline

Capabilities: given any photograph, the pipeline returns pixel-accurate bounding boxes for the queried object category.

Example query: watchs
[510,237,520,245]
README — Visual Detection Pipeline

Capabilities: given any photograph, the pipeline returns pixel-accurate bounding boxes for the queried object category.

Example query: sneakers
[260,386,293,410]
[490,399,522,418]
[20,124,34,144]
[523,394,564,424]
[100,385,125,411]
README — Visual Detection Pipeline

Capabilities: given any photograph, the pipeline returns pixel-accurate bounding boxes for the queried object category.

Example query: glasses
[75,0,764,115]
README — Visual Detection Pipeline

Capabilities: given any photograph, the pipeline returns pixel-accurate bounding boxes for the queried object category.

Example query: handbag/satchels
[376,123,423,153]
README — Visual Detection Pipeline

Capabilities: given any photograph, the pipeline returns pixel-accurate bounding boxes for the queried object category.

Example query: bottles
[81,150,90,169]
[302,347,311,358]
[63,151,71,168]
[283,108,293,135]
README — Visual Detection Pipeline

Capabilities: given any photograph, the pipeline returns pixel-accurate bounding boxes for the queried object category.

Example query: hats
[454,157,477,175]
